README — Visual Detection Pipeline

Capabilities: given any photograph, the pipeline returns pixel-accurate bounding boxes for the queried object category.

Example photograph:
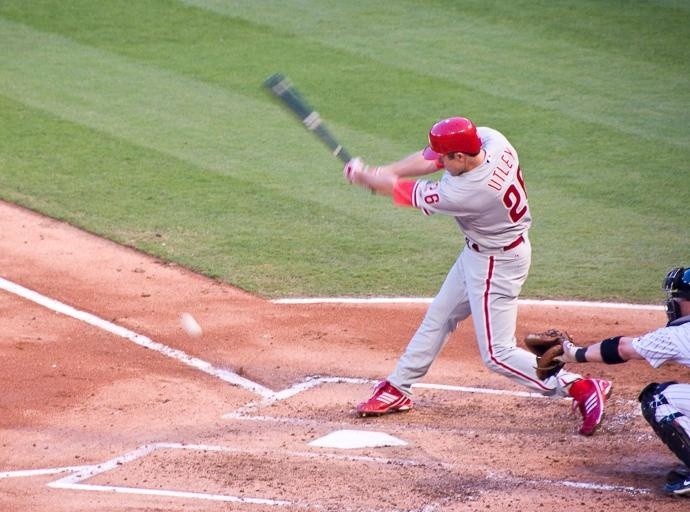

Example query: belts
[464,236,528,255]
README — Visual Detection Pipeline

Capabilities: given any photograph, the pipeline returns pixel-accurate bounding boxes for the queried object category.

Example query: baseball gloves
[524,329,574,381]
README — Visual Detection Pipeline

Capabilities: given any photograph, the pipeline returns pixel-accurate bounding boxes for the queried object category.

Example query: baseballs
[182,316,205,338]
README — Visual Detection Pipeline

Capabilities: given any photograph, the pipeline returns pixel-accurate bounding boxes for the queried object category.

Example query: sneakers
[662,471,690,496]
[357,379,416,419]
[569,373,614,436]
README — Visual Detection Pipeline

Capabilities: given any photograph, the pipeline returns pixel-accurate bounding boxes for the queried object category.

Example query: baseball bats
[265,72,381,196]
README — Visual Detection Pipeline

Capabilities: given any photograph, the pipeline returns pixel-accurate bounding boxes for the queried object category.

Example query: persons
[342,114,615,437]
[552,267,689,496]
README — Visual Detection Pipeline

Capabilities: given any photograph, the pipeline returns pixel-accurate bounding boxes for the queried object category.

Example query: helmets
[423,115,483,161]
[660,265,690,302]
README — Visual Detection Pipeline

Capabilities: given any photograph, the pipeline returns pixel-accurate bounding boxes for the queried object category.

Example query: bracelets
[574,346,590,364]
